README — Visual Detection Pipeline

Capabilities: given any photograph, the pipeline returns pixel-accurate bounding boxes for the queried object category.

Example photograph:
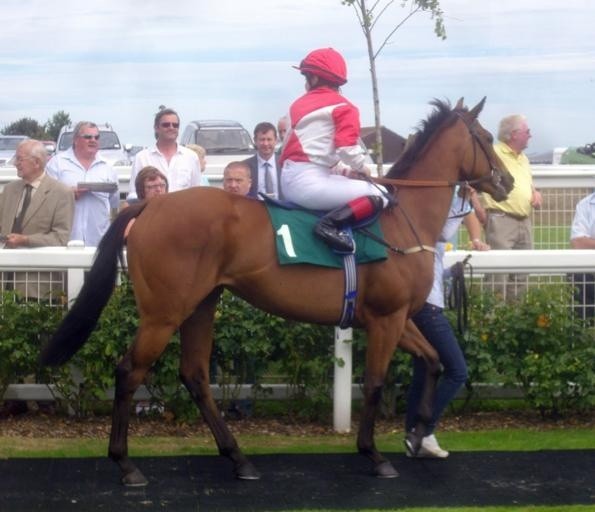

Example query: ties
[263,162,274,195]
[16,184,32,239]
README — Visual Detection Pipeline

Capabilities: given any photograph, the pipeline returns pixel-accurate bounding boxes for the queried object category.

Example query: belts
[486,208,529,221]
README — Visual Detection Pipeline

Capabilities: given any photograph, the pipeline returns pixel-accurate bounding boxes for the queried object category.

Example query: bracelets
[471,238,480,242]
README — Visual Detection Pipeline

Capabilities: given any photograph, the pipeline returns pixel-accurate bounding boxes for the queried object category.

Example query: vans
[180,117,257,188]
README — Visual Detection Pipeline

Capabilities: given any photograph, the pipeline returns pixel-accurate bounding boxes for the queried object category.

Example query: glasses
[161,122,180,128]
[77,134,101,141]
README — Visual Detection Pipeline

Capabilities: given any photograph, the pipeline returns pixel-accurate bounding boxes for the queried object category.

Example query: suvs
[56,120,134,167]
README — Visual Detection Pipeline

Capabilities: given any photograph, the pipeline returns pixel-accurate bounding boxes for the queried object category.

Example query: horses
[34,95,517,489]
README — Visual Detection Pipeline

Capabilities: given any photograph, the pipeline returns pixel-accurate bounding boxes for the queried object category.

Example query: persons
[566,192,594,326]
[45,120,120,246]
[277,116,288,155]
[184,143,211,187]
[463,113,543,297]
[241,122,283,201]
[403,182,472,459]
[280,46,389,253]
[126,105,203,200]
[222,160,252,197]
[119,166,168,242]
[464,213,491,250]
[1,139,75,307]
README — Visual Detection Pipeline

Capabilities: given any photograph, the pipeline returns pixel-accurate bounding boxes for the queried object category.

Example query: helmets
[292,48,347,85]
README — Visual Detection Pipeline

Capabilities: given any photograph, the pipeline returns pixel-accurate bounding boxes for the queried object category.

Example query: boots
[315,195,383,252]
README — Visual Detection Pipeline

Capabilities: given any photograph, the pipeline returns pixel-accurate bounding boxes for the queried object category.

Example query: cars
[0,134,31,168]
[40,139,57,159]
[127,144,149,164]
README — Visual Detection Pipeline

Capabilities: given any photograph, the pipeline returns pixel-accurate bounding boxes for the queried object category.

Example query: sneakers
[406,434,449,460]
